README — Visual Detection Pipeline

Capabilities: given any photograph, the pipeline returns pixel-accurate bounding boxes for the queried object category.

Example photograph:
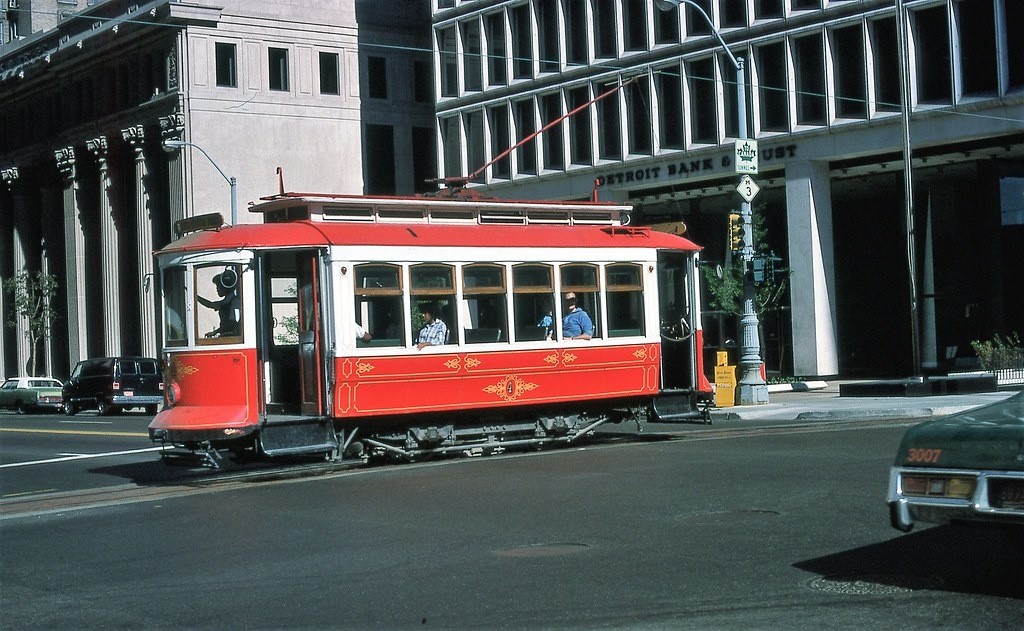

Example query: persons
[546,292,593,341]
[355,322,372,343]
[185,273,240,338]
[413,302,449,351]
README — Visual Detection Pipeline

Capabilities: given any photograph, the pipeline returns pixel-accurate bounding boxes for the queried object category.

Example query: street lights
[654,0,770,405]
[164,140,238,225]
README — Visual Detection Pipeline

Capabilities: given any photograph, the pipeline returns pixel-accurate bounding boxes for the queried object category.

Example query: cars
[886,388,1024,533]
[0,377,64,415]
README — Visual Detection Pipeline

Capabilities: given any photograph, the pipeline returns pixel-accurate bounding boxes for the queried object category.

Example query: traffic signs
[734,138,758,174]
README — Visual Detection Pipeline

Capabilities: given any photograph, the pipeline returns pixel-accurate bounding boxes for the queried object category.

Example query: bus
[147,76,724,474]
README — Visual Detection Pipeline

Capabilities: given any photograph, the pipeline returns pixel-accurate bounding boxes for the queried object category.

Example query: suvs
[61,356,165,416]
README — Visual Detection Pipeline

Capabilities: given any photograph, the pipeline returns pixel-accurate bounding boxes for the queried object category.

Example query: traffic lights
[768,257,786,283]
[729,214,745,251]
[747,259,765,282]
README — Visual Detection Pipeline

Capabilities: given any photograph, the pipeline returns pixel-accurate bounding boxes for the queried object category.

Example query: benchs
[356,327,640,347]
